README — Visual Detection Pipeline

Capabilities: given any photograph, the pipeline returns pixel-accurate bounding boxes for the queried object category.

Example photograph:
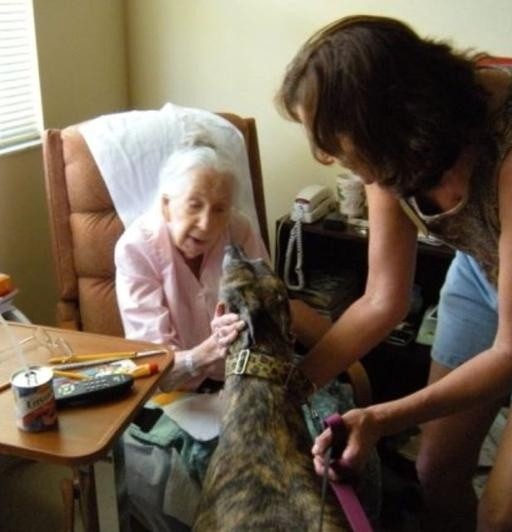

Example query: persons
[113,145,272,393]
[279,15,512,532]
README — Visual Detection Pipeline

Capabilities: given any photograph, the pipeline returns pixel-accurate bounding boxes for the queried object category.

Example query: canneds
[9,365,59,433]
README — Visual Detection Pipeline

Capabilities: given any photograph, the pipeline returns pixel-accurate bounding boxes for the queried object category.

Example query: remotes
[55,372,137,403]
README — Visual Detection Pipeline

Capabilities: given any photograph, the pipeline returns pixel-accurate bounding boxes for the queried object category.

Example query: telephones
[283,182,338,292]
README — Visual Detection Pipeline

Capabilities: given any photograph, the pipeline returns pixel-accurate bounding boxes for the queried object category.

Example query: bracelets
[184,349,200,379]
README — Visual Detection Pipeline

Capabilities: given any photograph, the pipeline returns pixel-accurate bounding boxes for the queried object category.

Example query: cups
[336,173,365,217]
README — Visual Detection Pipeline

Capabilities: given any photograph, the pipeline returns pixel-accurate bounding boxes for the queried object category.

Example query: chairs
[34,112,373,502]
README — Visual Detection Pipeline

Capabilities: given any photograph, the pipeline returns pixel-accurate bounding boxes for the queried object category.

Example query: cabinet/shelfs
[276,205,470,416]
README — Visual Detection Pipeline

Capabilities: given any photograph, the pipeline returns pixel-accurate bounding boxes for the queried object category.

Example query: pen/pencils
[48,351,137,364]
[52,369,85,385]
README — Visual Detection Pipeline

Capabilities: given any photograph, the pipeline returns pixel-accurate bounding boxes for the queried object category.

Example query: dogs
[189,241,352,532]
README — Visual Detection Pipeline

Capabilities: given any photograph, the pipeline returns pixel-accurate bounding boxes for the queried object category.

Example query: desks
[2,316,173,532]
